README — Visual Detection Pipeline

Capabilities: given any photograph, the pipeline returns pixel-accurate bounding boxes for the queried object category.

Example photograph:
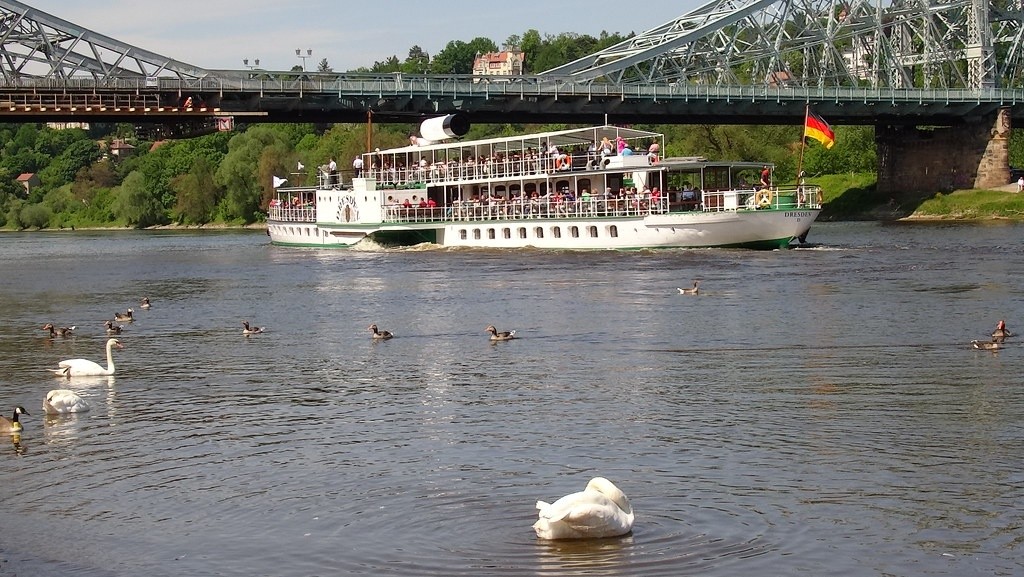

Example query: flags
[806,105,834,149]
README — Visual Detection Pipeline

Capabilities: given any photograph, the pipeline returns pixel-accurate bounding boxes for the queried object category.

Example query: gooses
[676,279,703,295]
[0,405,33,433]
[968,319,1010,351]
[140,299,153,309]
[47,338,125,377]
[104,319,124,336]
[113,308,135,325]
[533,474,634,541]
[484,324,515,341]
[44,323,76,336]
[42,388,92,415]
[242,321,265,334]
[368,324,394,338]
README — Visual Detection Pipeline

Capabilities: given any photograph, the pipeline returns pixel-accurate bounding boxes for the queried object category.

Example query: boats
[264,109,825,248]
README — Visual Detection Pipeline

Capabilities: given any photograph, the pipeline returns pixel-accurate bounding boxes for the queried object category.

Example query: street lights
[242,57,260,79]
[295,47,312,71]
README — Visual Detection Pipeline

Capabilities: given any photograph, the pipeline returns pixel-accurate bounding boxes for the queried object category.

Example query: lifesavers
[556,153,571,171]
[816,186,823,205]
[646,153,658,166]
[755,189,772,207]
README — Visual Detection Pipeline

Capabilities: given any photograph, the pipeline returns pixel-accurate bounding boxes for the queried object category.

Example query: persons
[184,97,192,107]
[650,140,659,156]
[270,195,306,209]
[761,166,770,190]
[670,183,701,210]
[1018,177,1024,190]
[620,144,638,155]
[739,174,748,187]
[372,142,559,183]
[403,197,436,221]
[329,157,337,187]
[588,140,594,154]
[447,186,665,221]
[598,137,613,154]
[353,155,362,177]
